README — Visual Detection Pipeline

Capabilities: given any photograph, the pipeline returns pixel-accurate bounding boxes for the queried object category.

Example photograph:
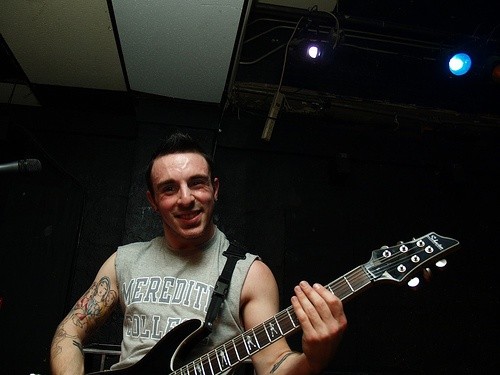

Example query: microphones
[0,158,41,173]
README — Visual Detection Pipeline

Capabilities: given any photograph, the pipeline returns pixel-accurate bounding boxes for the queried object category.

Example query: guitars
[83,230,462,375]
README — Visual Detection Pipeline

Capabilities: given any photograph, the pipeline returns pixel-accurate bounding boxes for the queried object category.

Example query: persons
[50,129,348,375]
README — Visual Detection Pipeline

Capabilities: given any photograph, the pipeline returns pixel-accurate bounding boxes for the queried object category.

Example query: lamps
[429,45,472,78]
[280,8,330,88]
